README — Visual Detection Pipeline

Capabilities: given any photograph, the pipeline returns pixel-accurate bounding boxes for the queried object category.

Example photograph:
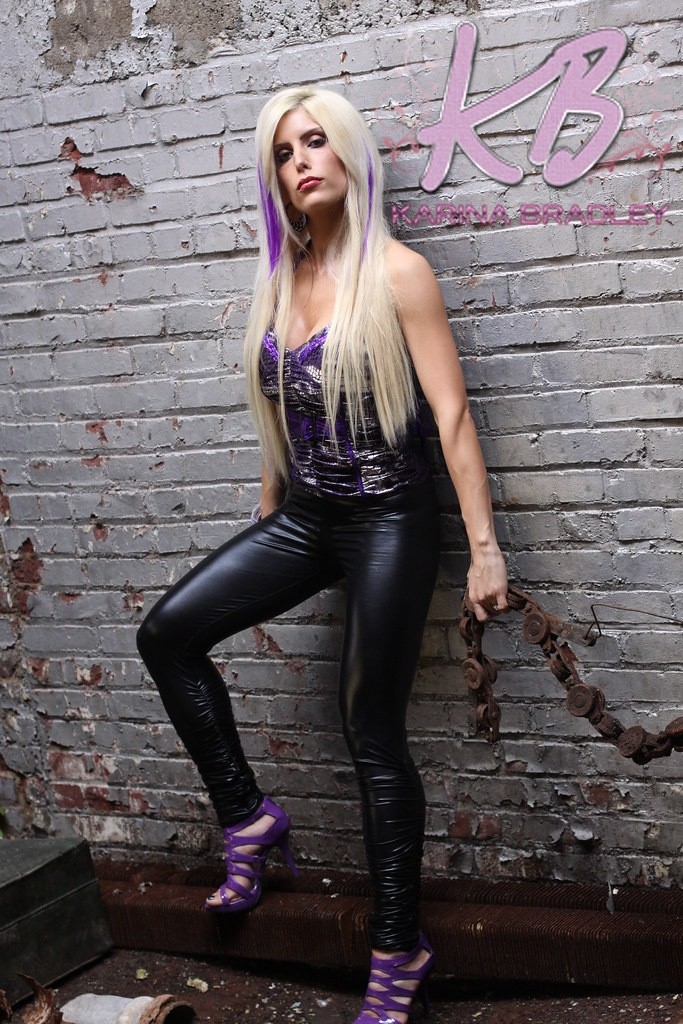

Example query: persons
[133,85,514,1024]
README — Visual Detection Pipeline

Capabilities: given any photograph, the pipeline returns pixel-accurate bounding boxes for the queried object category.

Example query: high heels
[351,930,436,1024]
[204,795,300,913]
